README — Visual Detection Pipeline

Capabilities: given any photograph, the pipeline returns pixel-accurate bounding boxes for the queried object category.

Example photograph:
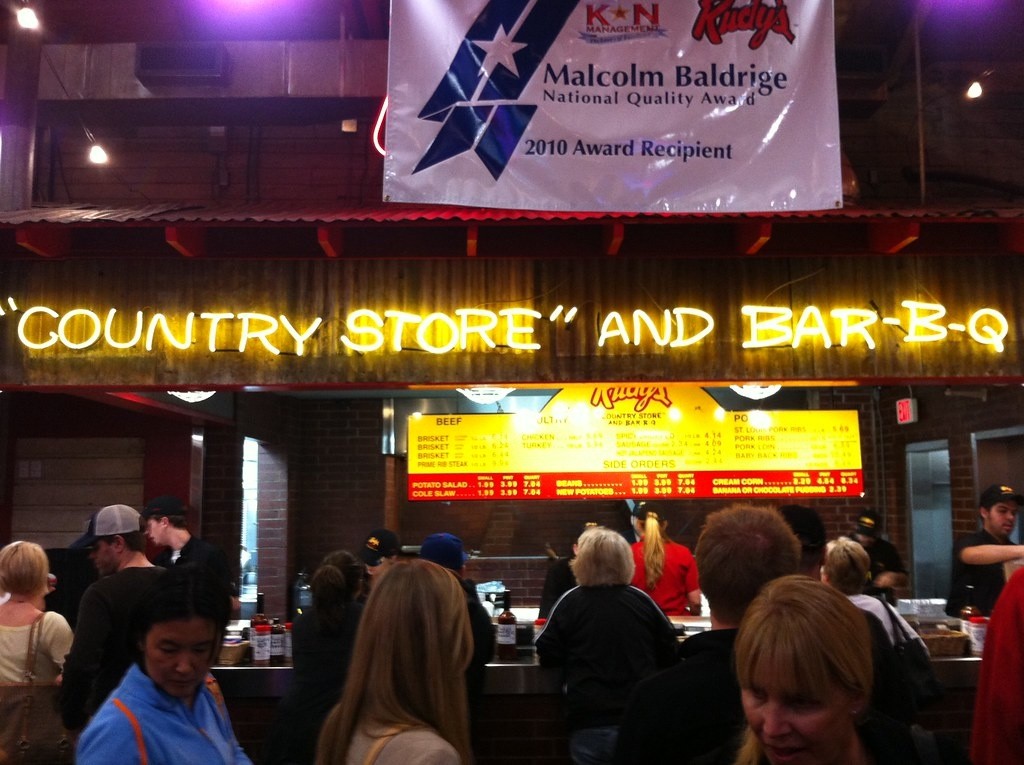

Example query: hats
[420,532,469,571]
[979,484,1024,508]
[630,501,666,523]
[855,509,883,536]
[69,504,142,549]
[144,495,187,515]
[356,528,398,564]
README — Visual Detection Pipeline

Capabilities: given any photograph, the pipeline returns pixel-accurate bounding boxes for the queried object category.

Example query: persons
[52,501,172,736]
[610,500,803,765]
[945,485,1024,623]
[969,564,1024,765]
[75,559,255,765]
[415,529,496,765]
[142,491,241,620]
[835,506,905,612]
[0,539,76,765]
[821,533,948,765]
[310,556,480,765]
[688,574,907,765]
[537,507,609,619]
[533,522,680,765]
[272,526,400,765]
[624,497,703,616]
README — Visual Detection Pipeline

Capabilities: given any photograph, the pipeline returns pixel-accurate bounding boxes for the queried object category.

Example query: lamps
[168,391,216,406]
[458,388,515,404]
[730,384,782,400]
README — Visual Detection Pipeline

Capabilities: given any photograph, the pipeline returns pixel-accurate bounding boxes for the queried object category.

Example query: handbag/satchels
[0,612,78,765]
[872,595,940,700]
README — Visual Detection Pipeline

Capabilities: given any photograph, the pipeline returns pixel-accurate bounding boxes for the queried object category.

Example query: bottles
[960,585,980,655]
[496,589,517,663]
[249,593,270,663]
